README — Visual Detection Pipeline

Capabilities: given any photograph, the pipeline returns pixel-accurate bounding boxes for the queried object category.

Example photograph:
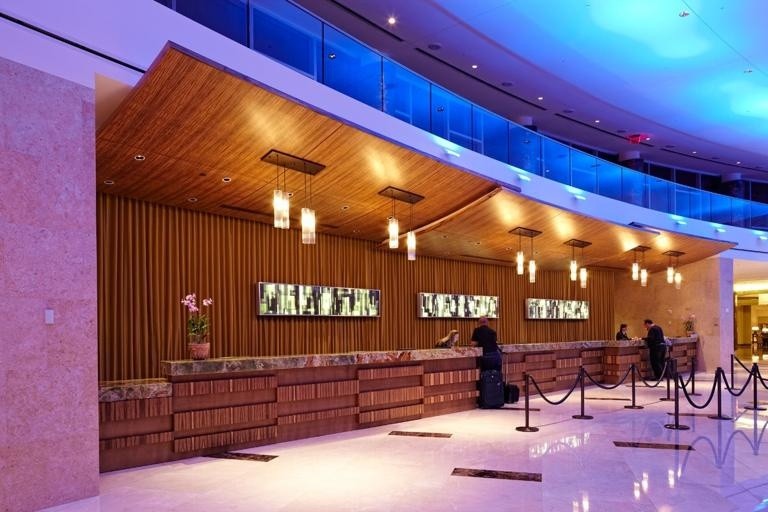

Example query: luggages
[480,369,505,408]
[664,351,677,378]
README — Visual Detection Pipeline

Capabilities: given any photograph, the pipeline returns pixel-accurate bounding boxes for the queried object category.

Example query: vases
[189,344,210,360]
[686,330,695,337]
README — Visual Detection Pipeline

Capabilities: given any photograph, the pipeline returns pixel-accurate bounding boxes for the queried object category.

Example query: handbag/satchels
[506,383,519,404]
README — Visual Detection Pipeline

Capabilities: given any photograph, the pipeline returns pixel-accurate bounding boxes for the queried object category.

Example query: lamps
[564,239,592,289]
[508,227,543,283]
[378,185,425,261]
[261,149,327,245]
[630,245,686,289]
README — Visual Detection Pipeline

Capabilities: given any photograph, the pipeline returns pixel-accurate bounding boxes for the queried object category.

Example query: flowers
[679,314,697,330]
[179,293,213,343]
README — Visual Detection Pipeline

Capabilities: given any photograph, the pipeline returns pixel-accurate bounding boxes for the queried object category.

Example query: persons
[639,319,667,381]
[471,317,502,372]
[436,329,460,348]
[616,324,628,340]
